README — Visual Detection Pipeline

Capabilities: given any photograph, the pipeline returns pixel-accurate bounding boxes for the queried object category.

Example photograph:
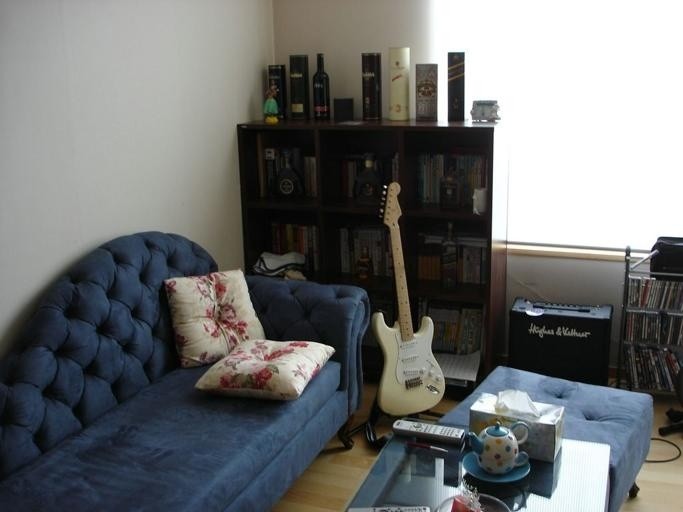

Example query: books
[624,276,683,392]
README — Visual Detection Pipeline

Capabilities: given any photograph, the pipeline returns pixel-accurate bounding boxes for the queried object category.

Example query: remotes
[348,507,431,512]
[393,420,465,445]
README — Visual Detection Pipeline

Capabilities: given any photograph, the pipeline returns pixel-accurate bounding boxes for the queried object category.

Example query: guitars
[371,183,445,415]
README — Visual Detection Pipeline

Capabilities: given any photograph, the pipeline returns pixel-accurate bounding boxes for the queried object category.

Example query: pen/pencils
[405,440,449,453]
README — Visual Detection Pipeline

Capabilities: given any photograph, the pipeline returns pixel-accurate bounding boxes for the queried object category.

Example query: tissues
[468,388,566,463]
[528,446,563,499]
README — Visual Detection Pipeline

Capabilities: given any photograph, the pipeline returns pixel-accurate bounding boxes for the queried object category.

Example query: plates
[433,492,513,512]
[460,452,532,484]
[463,472,535,501]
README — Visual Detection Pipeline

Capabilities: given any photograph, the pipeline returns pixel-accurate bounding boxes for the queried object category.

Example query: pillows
[160,266,266,370]
[193,335,336,405]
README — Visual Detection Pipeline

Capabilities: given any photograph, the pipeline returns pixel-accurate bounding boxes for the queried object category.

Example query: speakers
[508,296,614,386]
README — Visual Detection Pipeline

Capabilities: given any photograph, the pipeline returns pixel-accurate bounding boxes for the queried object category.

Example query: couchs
[0,230,373,510]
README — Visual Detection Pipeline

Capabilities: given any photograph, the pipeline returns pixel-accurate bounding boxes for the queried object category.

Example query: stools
[433,362,658,512]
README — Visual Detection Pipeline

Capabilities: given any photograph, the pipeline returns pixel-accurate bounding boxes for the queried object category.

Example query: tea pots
[461,477,526,512]
[462,418,530,477]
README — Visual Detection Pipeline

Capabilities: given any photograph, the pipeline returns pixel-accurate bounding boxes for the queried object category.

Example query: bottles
[312,52,331,121]
[356,245,375,279]
[352,157,384,208]
[436,159,463,211]
[438,222,459,293]
[270,150,305,202]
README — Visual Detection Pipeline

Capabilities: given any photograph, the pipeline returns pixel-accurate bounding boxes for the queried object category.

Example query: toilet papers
[472,187,488,216]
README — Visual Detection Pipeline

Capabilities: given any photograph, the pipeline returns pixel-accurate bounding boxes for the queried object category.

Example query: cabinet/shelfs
[615,246,682,405]
[235,117,510,402]
